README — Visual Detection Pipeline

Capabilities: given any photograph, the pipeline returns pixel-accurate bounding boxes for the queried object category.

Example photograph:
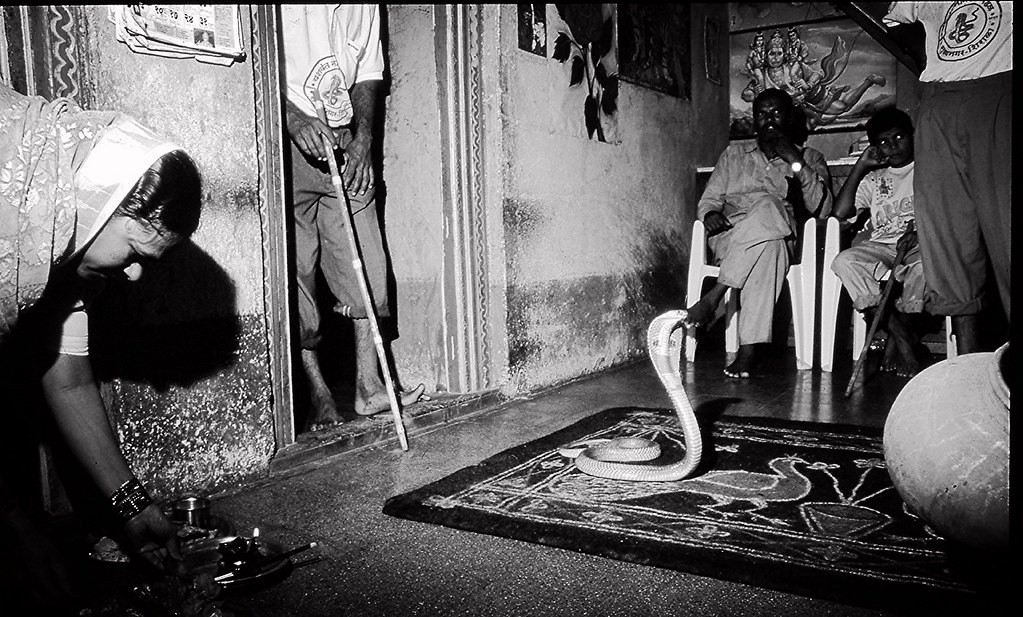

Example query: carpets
[383,407,1004,617]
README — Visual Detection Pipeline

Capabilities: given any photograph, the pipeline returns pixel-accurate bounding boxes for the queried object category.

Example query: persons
[0,81,200,572]
[883,1,1013,354]
[278,4,423,430]
[679,89,832,378]
[834,107,927,379]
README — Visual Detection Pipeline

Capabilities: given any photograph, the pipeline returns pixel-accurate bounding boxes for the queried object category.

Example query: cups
[172,497,211,532]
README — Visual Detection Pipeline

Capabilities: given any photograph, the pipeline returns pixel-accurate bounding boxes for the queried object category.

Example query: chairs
[822,216,958,368]
[684,217,819,367]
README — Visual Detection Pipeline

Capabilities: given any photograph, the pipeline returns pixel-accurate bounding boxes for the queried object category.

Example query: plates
[200,535,292,586]
[90,515,219,564]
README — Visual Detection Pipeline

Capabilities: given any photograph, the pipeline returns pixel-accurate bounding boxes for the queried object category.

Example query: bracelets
[109,478,153,521]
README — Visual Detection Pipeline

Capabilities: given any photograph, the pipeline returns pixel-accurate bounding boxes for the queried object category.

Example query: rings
[767,126,774,129]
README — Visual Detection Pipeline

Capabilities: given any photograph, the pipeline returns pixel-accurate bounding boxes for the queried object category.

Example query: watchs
[789,162,806,173]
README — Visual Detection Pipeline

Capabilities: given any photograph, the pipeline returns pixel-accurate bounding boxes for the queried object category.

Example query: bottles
[881,337,1016,544]
